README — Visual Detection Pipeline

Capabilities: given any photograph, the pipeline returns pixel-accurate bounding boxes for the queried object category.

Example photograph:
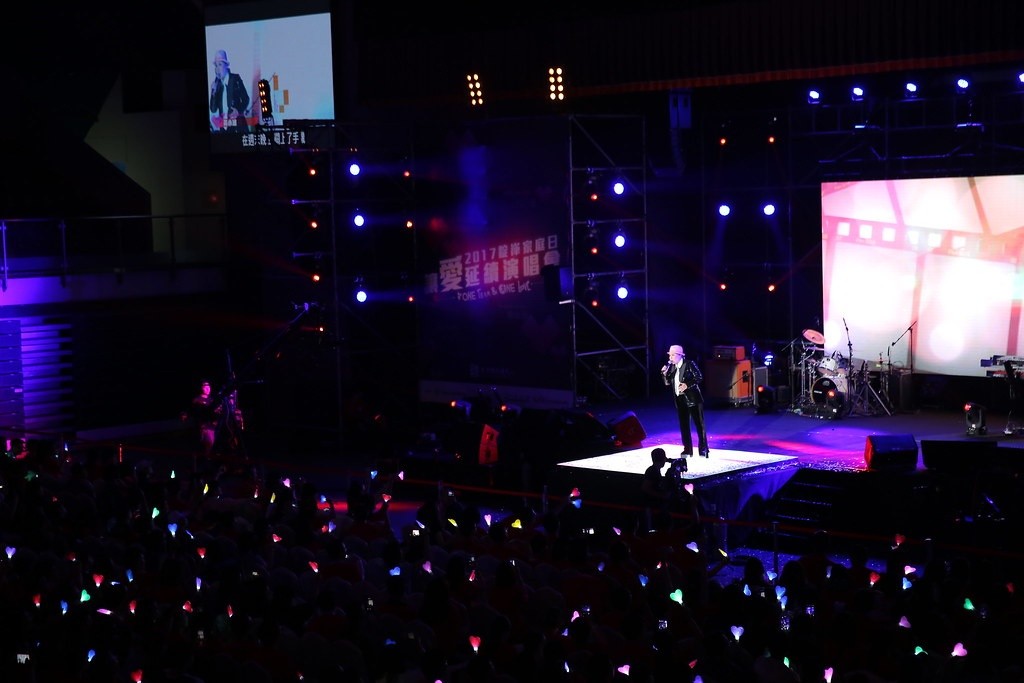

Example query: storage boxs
[752,366,769,406]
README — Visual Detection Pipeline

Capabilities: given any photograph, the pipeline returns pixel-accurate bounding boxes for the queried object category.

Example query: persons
[210,50,251,133]
[661,345,710,457]
[0,383,1024,683]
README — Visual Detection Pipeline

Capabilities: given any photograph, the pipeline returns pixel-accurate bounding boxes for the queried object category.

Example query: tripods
[786,343,816,412]
[841,320,895,416]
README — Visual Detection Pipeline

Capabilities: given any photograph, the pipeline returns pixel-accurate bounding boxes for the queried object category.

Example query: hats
[667,345,685,356]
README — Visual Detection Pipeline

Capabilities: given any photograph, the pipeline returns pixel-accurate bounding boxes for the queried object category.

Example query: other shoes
[681,446,693,457]
[699,447,709,458]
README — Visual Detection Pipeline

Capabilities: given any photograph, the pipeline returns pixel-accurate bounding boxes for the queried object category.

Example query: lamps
[756,385,777,413]
[823,389,846,419]
[963,403,988,436]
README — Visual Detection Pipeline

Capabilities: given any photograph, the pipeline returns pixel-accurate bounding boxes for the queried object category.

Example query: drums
[837,358,854,376]
[817,355,840,376]
[810,375,854,406]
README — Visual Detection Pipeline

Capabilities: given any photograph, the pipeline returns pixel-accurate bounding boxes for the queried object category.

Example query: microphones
[661,361,672,374]
[817,318,819,326]
[211,75,219,93]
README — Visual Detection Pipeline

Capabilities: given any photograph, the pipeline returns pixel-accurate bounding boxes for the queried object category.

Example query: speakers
[864,434,919,469]
[518,408,553,420]
[566,407,648,445]
[704,359,752,399]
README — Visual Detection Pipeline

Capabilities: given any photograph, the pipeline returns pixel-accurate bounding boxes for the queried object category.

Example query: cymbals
[805,347,824,351]
[802,329,824,345]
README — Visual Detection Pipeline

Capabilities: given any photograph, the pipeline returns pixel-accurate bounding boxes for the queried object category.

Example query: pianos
[979,354,1024,435]
